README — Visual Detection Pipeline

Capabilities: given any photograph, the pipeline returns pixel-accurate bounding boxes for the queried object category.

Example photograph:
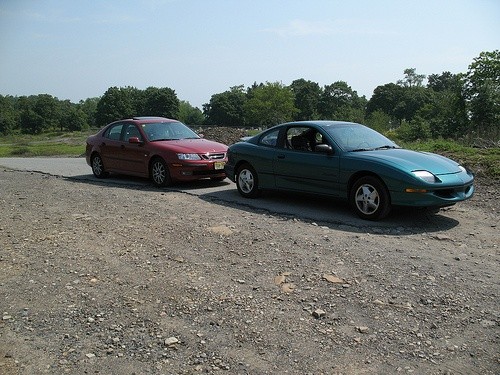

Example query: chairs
[309,132,326,152]
[291,135,309,151]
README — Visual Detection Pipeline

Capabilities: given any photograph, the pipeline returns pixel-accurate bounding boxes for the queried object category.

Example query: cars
[223,121,474,220]
[86,117,230,187]
